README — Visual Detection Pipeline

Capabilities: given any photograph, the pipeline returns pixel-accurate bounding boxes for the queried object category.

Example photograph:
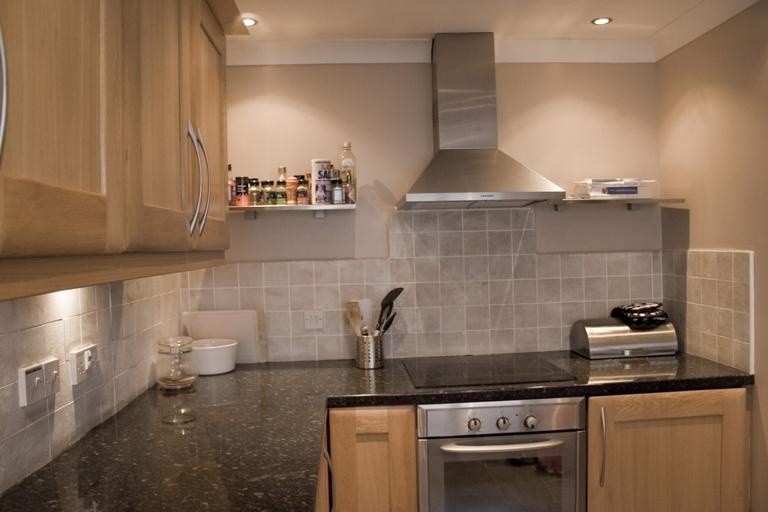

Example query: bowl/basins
[189,338,236,377]
[195,372,242,408]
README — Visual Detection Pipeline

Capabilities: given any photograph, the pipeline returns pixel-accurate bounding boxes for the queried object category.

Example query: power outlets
[70,344,101,386]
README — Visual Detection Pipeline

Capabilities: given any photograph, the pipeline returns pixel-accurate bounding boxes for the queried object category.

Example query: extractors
[392,33,567,211]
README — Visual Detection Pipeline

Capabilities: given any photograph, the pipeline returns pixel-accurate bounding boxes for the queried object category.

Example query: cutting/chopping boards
[175,310,267,367]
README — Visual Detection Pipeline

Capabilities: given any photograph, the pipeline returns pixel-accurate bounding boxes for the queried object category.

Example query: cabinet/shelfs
[583,385,756,512]
[316,426,332,510]
[110,0,232,259]
[330,401,419,511]
[1,1,118,263]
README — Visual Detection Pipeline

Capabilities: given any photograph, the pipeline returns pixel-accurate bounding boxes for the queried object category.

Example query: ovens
[415,428,585,511]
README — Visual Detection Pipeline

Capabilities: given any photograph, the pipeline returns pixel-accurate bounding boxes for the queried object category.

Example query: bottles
[227,141,357,207]
[150,335,200,390]
[157,387,198,432]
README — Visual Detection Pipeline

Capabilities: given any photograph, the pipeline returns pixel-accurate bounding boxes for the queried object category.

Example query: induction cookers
[401,353,587,438]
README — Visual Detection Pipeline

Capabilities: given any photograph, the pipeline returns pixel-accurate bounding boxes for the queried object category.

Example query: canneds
[235,177,286,207]
[296,179,309,205]
